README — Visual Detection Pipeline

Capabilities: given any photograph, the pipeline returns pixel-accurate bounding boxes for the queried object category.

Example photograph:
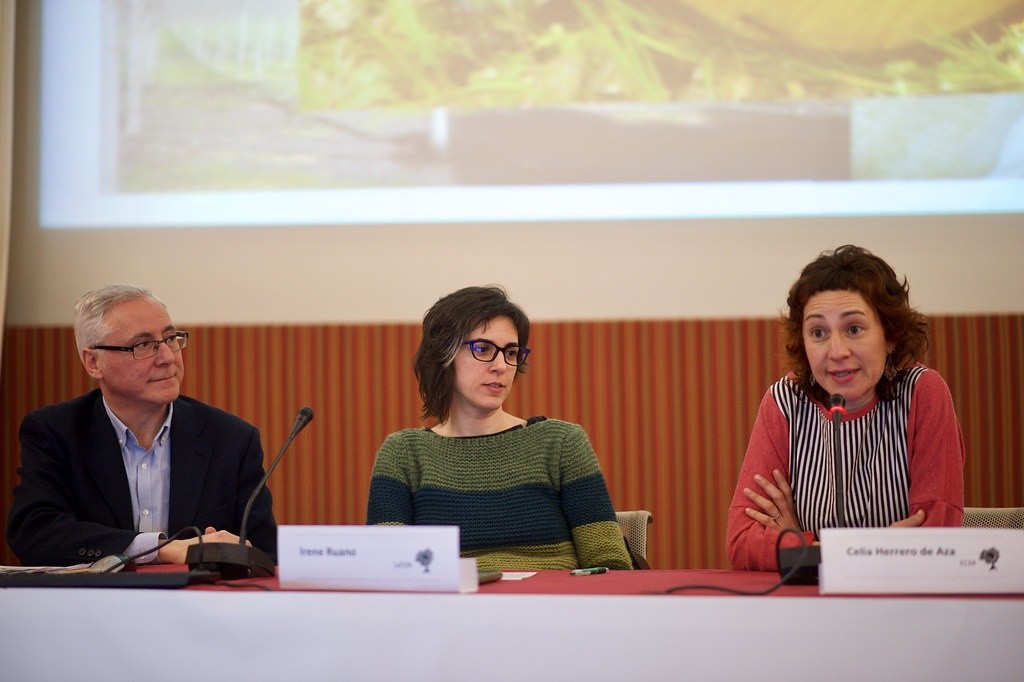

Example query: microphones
[778,393,850,584]
[183,405,315,579]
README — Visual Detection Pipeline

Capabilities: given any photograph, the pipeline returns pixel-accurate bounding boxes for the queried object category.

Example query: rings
[773,513,780,521]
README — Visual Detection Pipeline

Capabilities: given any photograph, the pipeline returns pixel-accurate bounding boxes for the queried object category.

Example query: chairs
[616,509,652,567]
[962,507,1024,530]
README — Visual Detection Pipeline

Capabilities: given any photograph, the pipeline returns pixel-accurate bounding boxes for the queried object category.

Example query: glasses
[89,331,190,361]
[462,339,530,367]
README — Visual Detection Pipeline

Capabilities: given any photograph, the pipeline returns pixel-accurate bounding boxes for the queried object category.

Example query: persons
[367,286,633,571]
[725,244,964,570]
[7,284,277,570]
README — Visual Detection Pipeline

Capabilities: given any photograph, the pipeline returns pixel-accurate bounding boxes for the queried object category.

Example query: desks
[0,566,1024,682]
